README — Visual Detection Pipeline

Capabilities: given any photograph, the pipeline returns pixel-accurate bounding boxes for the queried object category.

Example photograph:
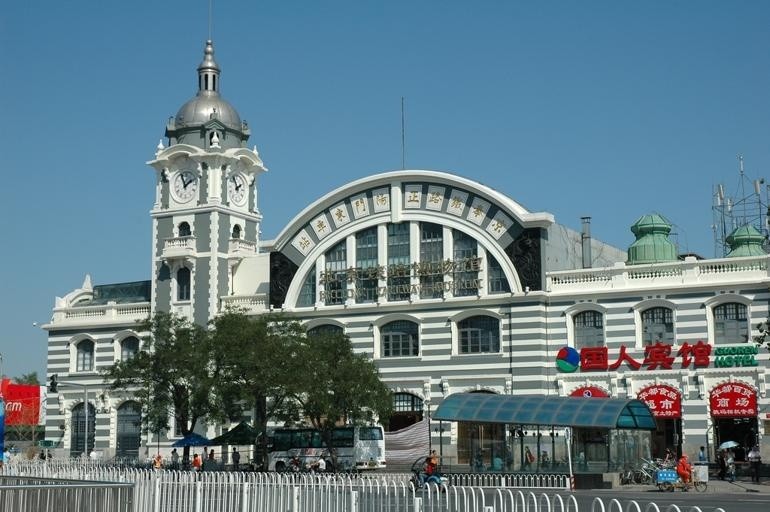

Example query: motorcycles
[409,456,455,493]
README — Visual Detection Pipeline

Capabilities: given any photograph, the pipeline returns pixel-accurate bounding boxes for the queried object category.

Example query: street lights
[425,399,432,456]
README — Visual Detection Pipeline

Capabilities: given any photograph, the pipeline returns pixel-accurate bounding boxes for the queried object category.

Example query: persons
[677,452,693,484]
[209,449,214,458]
[522,445,535,472]
[89,446,97,460]
[171,450,174,467]
[474,448,485,471]
[204,453,221,471]
[699,445,707,461]
[3,446,10,466]
[47,449,53,458]
[152,453,162,472]
[727,449,736,474]
[309,454,327,485]
[419,457,433,487]
[748,446,761,481]
[540,449,549,470]
[201,446,208,470]
[431,449,440,472]
[40,448,46,460]
[504,445,513,472]
[191,453,200,472]
[232,446,240,469]
[172,448,179,465]
[718,448,728,479]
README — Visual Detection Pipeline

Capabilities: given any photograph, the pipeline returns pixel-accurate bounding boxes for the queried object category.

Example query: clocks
[169,169,250,207]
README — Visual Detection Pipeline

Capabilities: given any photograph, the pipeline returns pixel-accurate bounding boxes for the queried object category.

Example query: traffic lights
[51,375,58,393]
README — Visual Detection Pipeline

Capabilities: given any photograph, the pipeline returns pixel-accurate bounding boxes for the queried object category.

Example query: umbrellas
[208,420,259,455]
[172,432,209,456]
[719,441,740,449]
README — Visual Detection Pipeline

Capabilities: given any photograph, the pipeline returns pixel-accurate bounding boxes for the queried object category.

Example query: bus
[253,423,386,472]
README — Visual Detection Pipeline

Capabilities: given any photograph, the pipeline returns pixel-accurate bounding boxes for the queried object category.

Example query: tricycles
[653,468,707,493]
